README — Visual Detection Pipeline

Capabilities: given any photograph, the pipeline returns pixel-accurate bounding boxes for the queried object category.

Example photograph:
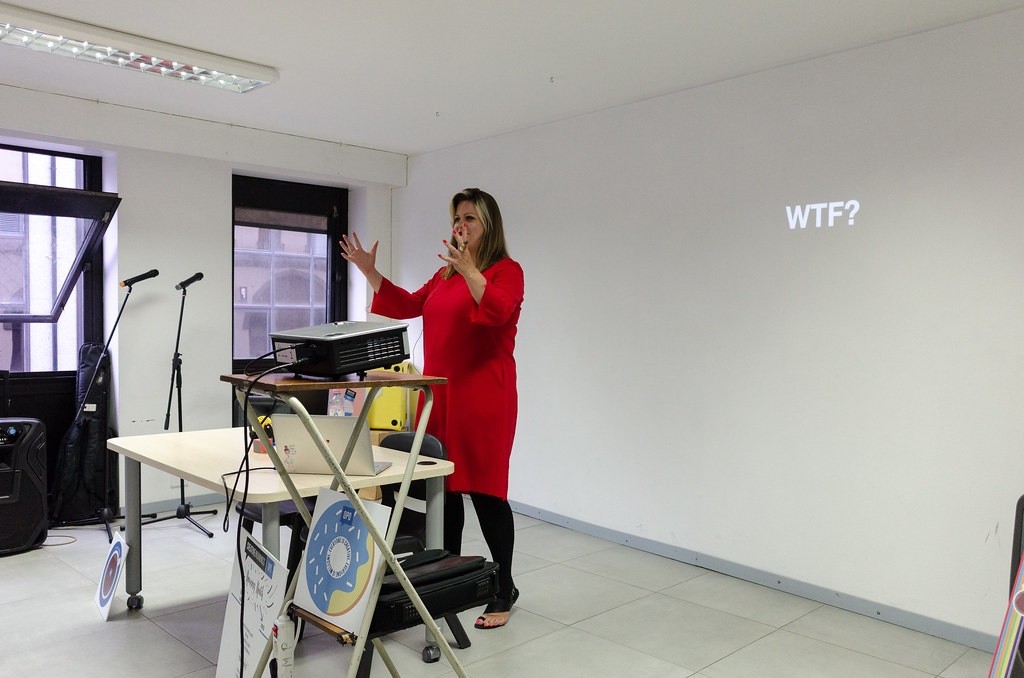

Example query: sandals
[475,587,520,629]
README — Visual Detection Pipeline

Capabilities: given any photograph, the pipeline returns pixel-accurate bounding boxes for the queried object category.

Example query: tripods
[58,285,157,543]
[120,288,217,538]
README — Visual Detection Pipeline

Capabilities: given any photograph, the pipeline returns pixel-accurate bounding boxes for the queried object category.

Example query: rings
[350,252,355,257]
[459,240,469,253]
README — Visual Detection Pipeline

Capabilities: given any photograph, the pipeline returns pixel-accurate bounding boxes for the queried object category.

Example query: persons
[339,186,525,629]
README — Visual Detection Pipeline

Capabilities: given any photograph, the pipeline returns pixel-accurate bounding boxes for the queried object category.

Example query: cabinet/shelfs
[217,363,467,678]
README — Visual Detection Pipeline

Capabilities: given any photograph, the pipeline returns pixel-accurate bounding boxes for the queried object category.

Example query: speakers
[0,418,48,554]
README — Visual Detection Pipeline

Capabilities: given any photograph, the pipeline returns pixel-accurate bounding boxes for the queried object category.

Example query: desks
[104,425,455,678]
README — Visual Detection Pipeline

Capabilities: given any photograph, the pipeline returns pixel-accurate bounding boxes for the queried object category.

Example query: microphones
[175,272,204,290]
[120,269,159,287]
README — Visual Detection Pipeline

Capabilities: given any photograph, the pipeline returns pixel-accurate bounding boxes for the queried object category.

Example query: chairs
[234,496,317,599]
[303,432,473,651]
